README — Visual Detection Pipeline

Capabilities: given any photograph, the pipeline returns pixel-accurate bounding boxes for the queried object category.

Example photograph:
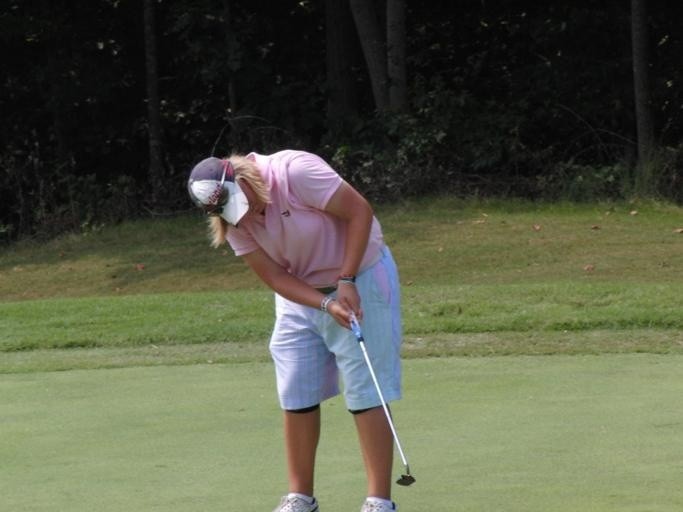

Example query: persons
[188,148,409,512]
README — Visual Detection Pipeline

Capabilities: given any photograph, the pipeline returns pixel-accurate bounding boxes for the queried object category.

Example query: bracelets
[319,294,331,313]
[336,273,354,280]
[336,272,354,283]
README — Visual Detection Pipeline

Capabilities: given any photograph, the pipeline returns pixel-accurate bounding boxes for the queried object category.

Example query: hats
[186,155,249,226]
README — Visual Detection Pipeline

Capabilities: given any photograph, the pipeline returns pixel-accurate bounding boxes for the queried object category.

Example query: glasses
[202,160,232,218]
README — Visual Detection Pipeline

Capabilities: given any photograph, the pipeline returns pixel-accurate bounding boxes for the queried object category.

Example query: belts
[313,247,384,294]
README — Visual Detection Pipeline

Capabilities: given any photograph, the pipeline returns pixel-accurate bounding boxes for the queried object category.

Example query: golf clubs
[348,310,415,486]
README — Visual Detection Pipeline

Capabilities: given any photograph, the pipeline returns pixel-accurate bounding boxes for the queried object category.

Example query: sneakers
[272,495,319,512]
[359,497,396,511]
